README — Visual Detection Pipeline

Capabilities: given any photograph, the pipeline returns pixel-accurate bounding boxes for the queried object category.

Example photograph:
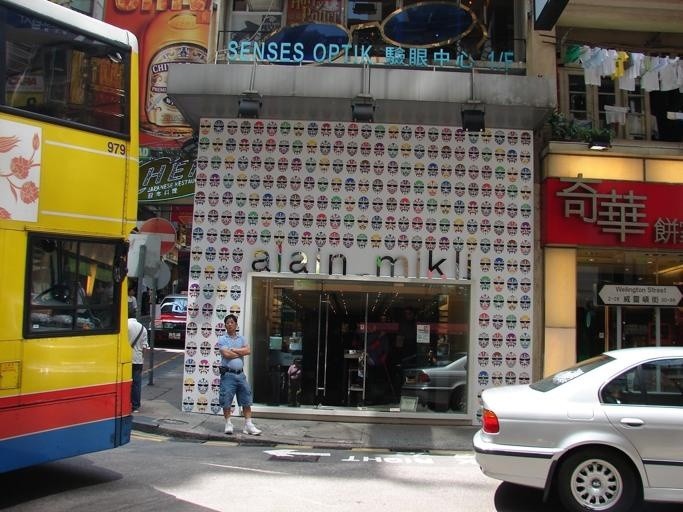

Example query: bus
[0,2,143,478]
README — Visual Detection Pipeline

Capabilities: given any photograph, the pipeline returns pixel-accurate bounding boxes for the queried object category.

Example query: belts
[226,366,243,375]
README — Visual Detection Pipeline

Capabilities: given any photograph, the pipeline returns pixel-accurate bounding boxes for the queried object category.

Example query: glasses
[252,1,490,68]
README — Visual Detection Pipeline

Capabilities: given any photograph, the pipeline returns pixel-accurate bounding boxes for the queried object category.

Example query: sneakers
[224,422,234,434]
[243,424,262,435]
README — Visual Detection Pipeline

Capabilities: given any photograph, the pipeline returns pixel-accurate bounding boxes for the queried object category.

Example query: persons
[287,358,302,407]
[128,287,136,316]
[128,305,148,411]
[216,314,262,435]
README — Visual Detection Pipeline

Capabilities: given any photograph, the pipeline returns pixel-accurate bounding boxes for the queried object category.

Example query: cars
[471,344,683,511]
[402,352,466,412]
[147,291,188,349]
